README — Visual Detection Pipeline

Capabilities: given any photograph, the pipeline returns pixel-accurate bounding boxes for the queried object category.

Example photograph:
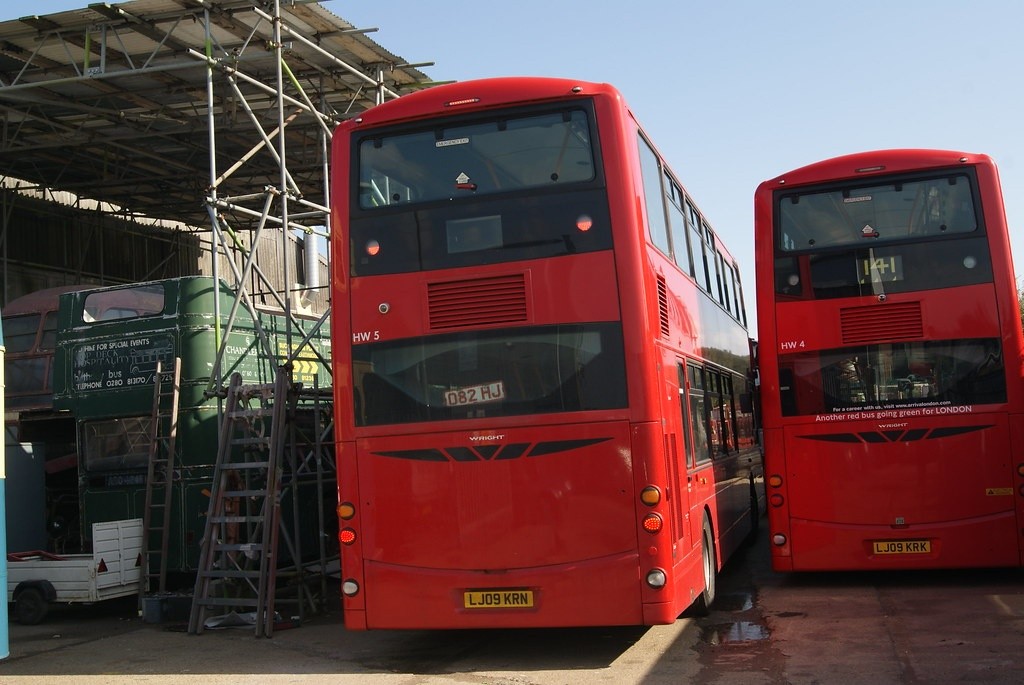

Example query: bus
[754,149,1024,571]
[331,77,768,632]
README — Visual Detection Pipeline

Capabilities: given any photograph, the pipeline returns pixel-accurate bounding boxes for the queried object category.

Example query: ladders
[137,355,182,617]
[188,367,289,639]
